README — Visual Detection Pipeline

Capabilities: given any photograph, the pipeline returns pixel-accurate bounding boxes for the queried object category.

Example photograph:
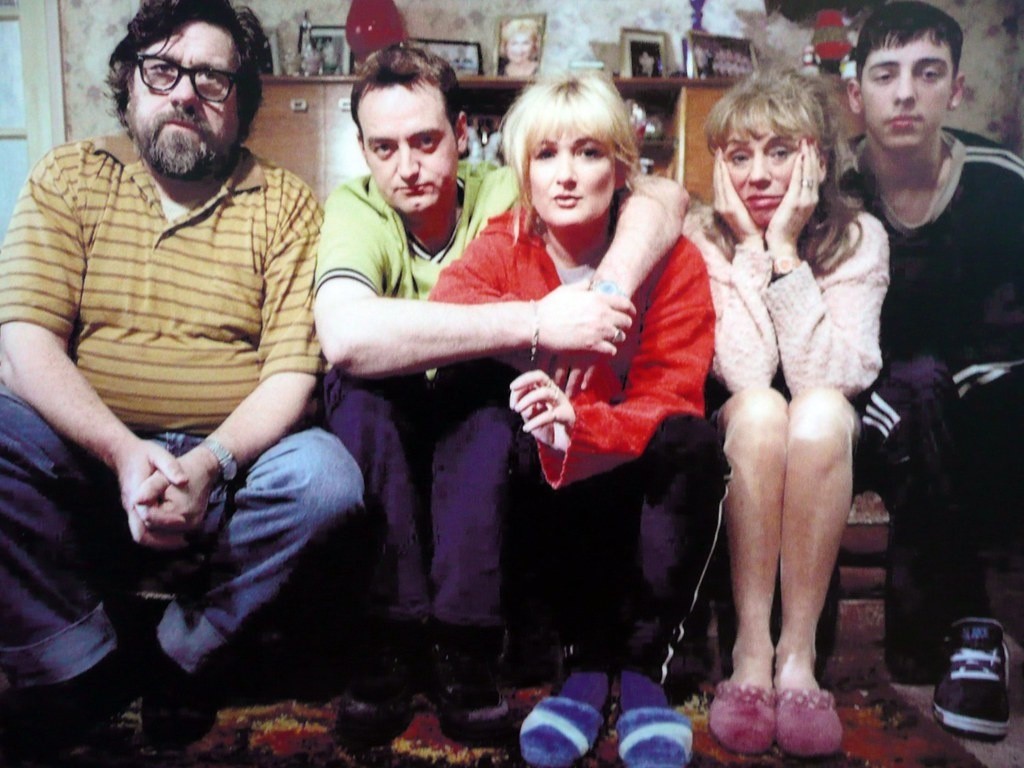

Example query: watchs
[203,437,237,486]
[588,279,627,299]
[772,258,801,276]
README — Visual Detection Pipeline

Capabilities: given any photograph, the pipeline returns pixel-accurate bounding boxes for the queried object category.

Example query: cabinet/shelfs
[241,79,740,207]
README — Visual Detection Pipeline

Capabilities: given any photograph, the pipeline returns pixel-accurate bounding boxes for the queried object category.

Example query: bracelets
[530,298,540,361]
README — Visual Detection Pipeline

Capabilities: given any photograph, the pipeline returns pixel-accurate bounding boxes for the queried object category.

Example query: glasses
[132,55,239,102]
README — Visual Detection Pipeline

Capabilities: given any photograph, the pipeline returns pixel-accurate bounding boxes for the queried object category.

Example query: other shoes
[431,629,513,743]
[141,664,216,752]
[0,662,134,755]
[338,635,415,744]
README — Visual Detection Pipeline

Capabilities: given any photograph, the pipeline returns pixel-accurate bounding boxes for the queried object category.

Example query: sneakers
[932,617,1010,737]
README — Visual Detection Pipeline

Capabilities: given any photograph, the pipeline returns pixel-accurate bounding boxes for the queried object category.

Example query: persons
[313,41,729,767]
[833,0,1023,736]
[0,0,364,768]
[693,42,753,78]
[304,38,337,76]
[682,69,890,756]
[441,24,539,78]
[639,50,652,78]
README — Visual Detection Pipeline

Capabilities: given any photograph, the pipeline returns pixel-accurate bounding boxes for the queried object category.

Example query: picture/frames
[492,12,547,77]
[407,38,484,75]
[620,27,667,78]
[688,31,761,81]
[298,25,355,77]
[245,28,282,76]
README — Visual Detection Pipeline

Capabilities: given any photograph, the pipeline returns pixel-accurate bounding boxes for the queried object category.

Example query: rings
[801,178,814,189]
[547,379,560,406]
[609,328,622,343]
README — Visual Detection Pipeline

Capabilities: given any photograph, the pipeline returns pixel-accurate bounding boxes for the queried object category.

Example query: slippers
[709,681,777,752]
[619,705,695,768]
[777,688,844,756]
[518,696,605,768]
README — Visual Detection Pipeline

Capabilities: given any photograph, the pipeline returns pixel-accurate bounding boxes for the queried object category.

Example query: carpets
[0,681,986,768]
